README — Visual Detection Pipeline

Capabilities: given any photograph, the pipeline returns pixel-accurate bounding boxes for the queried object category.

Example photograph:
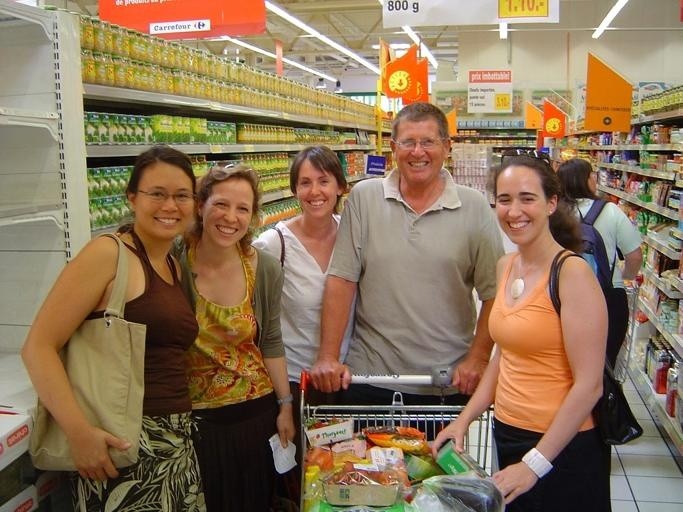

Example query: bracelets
[276,394,292,404]
[519,447,554,478]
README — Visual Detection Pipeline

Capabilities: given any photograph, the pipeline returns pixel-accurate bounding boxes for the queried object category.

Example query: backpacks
[576,197,621,292]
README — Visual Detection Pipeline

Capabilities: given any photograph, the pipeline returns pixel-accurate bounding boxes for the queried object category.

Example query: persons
[247,144,356,511]
[19,142,210,511]
[307,101,503,440]
[432,153,614,512]
[167,159,295,512]
[555,157,644,370]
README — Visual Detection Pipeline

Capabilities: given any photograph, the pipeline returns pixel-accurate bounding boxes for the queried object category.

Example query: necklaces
[509,246,547,300]
[407,181,440,213]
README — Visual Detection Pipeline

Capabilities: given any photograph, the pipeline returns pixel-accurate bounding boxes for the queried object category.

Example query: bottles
[644,332,682,424]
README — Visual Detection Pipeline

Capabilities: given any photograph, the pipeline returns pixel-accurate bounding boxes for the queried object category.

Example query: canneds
[44,6,375,194]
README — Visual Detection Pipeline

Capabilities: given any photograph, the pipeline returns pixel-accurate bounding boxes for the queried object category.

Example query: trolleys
[291,367,511,512]
[609,274,644,387]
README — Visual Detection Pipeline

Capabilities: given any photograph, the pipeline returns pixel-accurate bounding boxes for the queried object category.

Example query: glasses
[391,138,447,150]
[500,148,551,166]
[135,188,194,206]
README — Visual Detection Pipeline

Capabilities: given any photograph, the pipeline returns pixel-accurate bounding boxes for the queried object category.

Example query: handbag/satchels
[590,355,642,444]
[29,232,147,472]
